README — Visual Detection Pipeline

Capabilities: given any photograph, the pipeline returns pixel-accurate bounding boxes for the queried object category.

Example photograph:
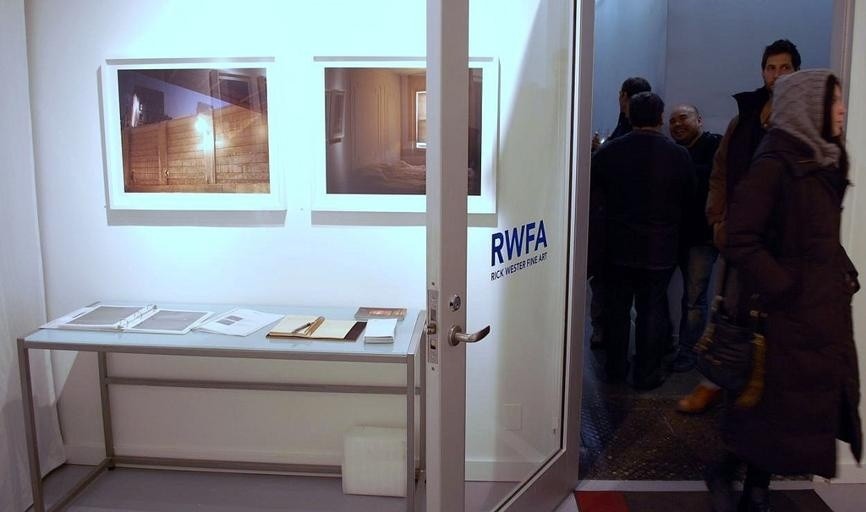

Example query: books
[191,304,408,345]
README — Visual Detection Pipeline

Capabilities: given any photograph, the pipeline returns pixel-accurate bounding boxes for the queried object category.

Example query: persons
[588,77,653,371]
[653,102,725,374]
[691,67,863,512]
[673,38,802,415]
[589,90,702,391]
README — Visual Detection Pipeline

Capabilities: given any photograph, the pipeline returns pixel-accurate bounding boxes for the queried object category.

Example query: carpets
[572,489,834,512]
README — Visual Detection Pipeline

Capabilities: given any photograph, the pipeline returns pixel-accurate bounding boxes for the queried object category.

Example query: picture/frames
[101,57,288,211]
[311,56,499,214]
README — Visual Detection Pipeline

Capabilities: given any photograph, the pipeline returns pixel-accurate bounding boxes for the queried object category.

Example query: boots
[736,336,766,411]
[702,451,775,512]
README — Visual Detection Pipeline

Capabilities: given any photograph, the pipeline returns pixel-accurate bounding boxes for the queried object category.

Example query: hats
[771,68,840,168]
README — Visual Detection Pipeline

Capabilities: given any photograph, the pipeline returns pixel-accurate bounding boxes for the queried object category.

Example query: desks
[17,301,427,512]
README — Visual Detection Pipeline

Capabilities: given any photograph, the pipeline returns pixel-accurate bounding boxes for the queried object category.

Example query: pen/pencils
[292,322,312,332]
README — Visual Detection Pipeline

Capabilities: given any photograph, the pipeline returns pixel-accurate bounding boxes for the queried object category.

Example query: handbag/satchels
[695,312,752,390]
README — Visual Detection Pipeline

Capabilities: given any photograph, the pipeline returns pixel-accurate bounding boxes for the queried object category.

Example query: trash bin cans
[340,425,408,497]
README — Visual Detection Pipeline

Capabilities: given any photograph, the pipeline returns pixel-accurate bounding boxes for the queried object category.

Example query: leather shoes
[677,385,724,414]
[635,375,664,390]
[607,376,624,383]
[669,349,697,371]
